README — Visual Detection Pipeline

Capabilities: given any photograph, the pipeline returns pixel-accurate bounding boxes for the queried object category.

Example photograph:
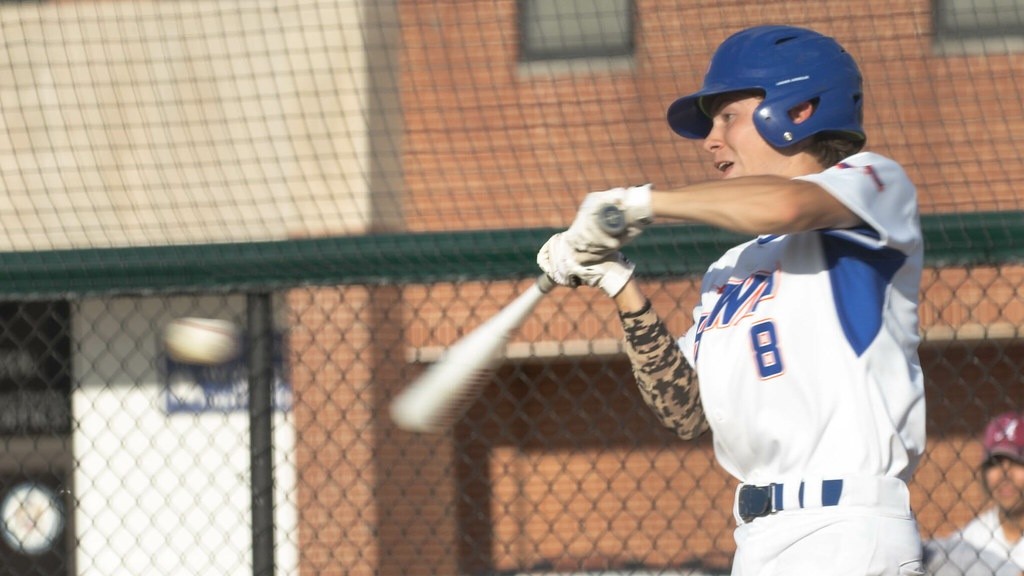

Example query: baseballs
[164,317,239,366]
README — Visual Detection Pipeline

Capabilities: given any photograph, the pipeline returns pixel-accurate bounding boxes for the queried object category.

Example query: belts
[739,479,843,522]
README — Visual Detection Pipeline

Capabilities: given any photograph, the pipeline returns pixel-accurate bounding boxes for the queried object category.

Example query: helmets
[980,412,1024,465]
[667,23,868,157]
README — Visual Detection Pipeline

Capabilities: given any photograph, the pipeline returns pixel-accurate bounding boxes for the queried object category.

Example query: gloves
[536,231,638,299]
[566,182,656,266]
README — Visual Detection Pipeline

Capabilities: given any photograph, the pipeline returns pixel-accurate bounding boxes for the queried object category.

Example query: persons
[927,413,1024,576]
[536,24,927,576]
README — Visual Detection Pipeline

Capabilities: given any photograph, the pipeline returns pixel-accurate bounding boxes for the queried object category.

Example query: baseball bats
[386,203,627,436]
